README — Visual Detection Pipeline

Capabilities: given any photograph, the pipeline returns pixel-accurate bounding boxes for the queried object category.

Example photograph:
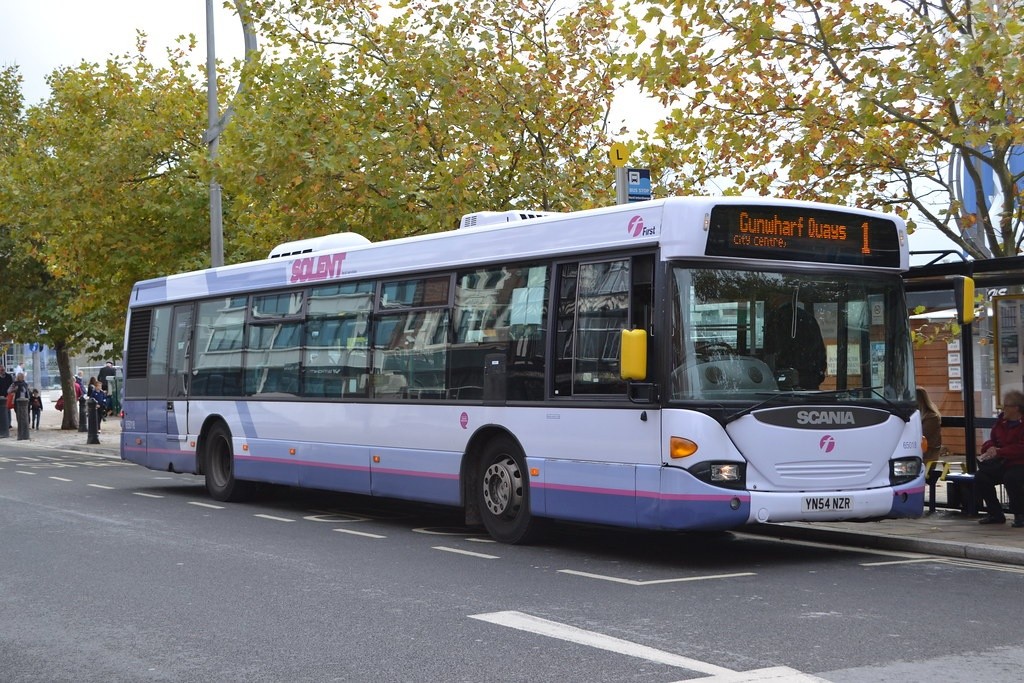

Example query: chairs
[183,345,442,400]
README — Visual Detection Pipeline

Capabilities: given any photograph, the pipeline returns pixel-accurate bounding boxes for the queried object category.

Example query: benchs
[946,474,980,516]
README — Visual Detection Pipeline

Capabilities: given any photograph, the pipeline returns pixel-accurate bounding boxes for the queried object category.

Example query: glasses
[1002,404,1018,410]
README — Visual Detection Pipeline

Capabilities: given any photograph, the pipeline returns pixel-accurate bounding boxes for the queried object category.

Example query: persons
[277,321,440,401]
[0,362,43,440]
[914,387,942,477]
[71,362,122,434]
[964,391,1024,527]
[761,285,825,391]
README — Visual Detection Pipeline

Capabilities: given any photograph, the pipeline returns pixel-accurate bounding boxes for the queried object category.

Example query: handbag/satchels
[55,396,64,411]
[7,393,14,409]
[977,458,1005,469]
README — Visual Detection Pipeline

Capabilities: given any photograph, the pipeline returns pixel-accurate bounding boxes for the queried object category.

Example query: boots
[1012,514,1024,527]
[979,501,1006,524]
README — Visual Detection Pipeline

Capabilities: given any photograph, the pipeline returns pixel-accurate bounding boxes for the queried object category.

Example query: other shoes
[98,429,101,433]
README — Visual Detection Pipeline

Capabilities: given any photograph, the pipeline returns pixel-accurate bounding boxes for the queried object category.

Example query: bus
[119,195,977,547]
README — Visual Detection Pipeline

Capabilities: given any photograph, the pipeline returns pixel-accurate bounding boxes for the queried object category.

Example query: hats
[106,361,113,364]
[765,281,793,295]
